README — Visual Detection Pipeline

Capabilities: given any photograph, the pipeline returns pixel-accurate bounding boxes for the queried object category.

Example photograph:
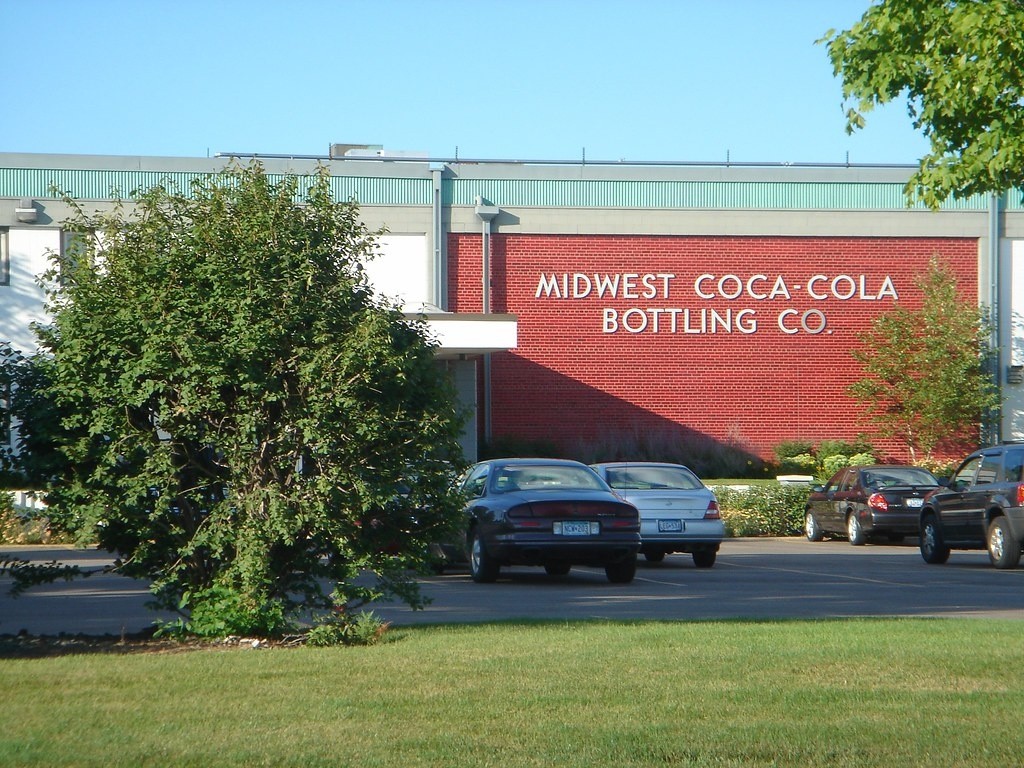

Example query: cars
[804,465,944,546]
[432,458,641,584]
[918,442,1024,568]
[587,462,726,568]
